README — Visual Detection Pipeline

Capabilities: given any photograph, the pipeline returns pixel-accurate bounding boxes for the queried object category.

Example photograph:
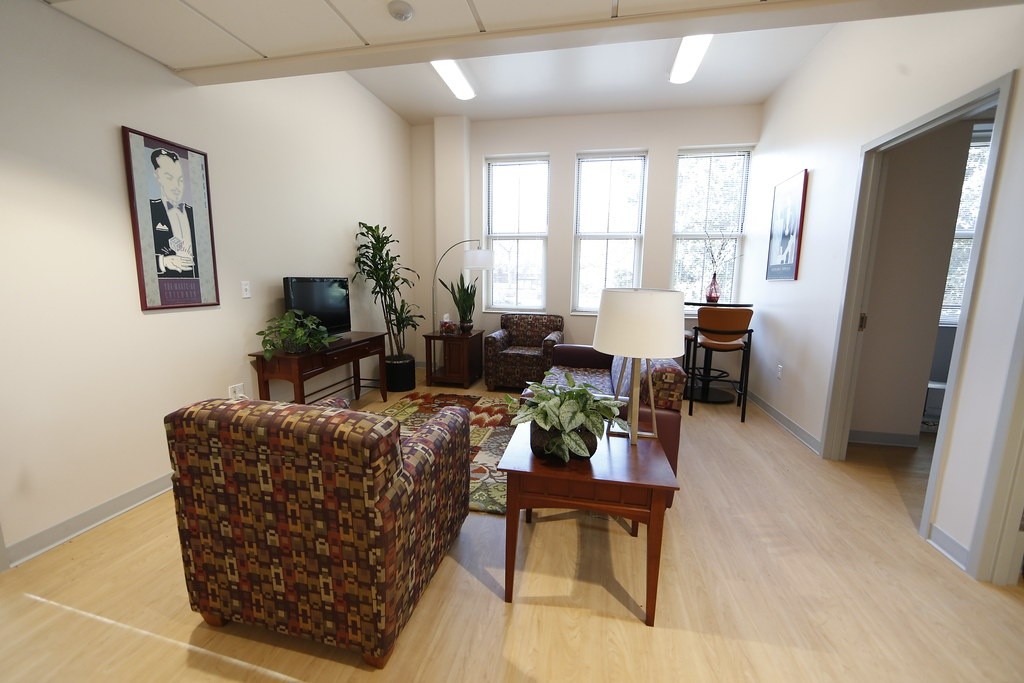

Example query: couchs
[484,313,564,391]
[163,398,469,670]
[520,344,687,508]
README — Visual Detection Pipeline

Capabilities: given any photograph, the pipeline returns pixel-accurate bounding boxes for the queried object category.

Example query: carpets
[378,392,520,515]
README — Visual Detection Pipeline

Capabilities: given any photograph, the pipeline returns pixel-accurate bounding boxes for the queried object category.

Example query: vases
[706,271,721,303]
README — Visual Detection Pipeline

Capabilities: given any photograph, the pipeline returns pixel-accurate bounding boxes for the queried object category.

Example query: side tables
[422,330,487,389]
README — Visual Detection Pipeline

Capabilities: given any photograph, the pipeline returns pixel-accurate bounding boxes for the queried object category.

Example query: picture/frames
[766,169,808,281]
[120,124,220,311]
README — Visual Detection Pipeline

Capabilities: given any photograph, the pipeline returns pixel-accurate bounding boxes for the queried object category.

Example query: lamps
[432,239,495,374]
[593,288,685,444]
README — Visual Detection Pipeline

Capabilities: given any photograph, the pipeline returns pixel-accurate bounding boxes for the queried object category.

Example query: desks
[248,331,389,404]
[684,302,754,404]
[497,421,680,627]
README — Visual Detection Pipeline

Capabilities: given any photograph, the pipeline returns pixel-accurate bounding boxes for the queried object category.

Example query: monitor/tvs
[283,276,351,338]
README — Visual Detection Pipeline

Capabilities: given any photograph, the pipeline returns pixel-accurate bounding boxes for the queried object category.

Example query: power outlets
[777,365,782,380]
[229,383,244,399]
[241,281,251,298]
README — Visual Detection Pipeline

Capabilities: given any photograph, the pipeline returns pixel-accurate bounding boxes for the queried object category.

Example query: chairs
[683,306,754,422]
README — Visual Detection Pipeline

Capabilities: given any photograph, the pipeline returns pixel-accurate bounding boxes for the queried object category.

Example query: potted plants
[504,372,631,468]
[351,222,426,392]
[438,273,478,332]
[256,309,342,362]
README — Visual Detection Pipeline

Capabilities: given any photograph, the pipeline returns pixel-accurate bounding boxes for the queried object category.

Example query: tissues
[439,313,452,335]
